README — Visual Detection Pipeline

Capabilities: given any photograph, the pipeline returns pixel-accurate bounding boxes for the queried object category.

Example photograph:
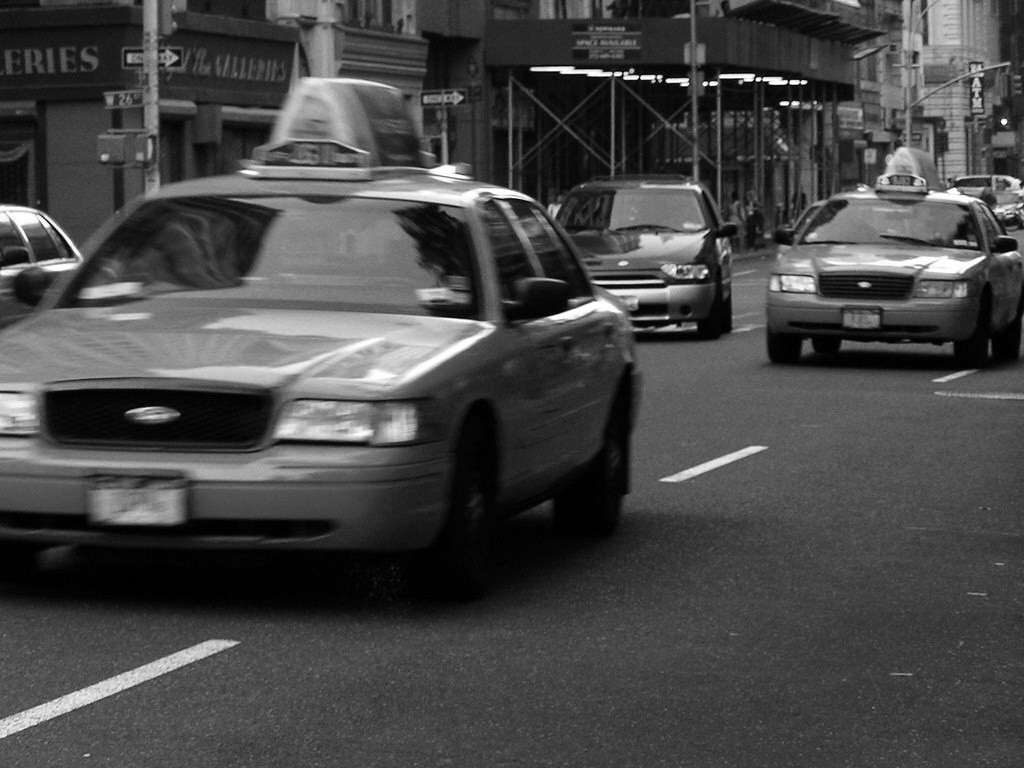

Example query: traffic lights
[1000,73,1023,98]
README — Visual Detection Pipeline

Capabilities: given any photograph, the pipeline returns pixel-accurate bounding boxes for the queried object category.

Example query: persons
[1017,181,1024,203]
[793,184,807,226]
[744,191,759,249]
[980,186,997,210]
[727,190,747,254]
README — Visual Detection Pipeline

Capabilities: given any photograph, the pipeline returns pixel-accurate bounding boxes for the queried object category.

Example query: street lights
[904,2,1012,146]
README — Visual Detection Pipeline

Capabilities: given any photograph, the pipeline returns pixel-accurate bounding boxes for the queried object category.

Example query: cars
[0,77,645,585]
[953,174,1024,230]
[764,148,1024,369]
[554,174,738,339]
